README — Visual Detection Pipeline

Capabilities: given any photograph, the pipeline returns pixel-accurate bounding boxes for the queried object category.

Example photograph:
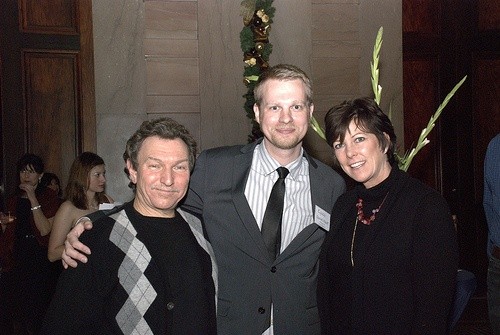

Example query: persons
[0,152,115,335]
[450,134,500,335]
[62,63,346,335]
[41,115,218,335]
[316,97,461,335]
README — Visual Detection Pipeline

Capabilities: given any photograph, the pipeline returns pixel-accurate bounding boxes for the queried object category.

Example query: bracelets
[31,205,42,210]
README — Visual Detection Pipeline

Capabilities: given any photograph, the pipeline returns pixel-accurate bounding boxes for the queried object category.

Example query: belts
[489,243,500,259]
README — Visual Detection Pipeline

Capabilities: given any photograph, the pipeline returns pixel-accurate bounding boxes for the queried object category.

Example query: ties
[260,166,289,258]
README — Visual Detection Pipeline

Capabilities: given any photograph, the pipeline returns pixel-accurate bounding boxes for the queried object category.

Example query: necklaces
[350,192,389,266]
[88,204,99,210]
